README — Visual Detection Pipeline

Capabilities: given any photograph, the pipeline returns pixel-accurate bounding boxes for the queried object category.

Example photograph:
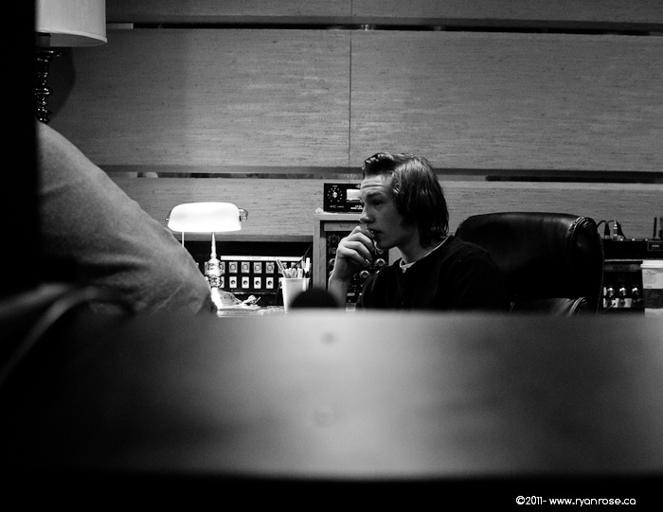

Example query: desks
[1,311,662,512]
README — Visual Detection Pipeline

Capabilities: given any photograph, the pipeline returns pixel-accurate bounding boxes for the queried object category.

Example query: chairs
[454,212,606,317]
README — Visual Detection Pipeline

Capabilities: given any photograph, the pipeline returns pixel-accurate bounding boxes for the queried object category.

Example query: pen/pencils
[276,259,304,278]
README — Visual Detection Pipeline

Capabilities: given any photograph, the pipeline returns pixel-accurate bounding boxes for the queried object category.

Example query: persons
[328,151,510,311]
[37,120,219,318]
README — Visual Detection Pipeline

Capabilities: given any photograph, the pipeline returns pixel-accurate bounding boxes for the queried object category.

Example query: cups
[639,259,663,317]
[278,278,310,313]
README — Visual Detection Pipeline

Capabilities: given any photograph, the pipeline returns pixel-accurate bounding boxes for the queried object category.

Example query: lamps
[167,202,247,294]
[33,1,109,124]
